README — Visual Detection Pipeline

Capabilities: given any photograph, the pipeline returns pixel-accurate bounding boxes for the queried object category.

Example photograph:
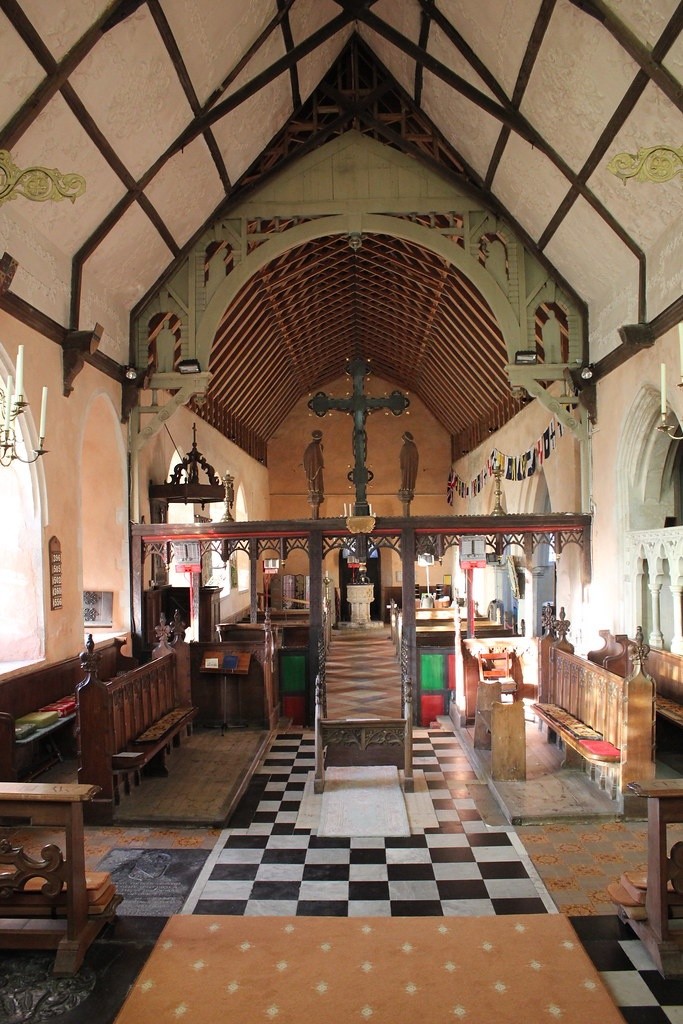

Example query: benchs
[530,606,683,980]
[0,633,193,972]
[220,599,331,660]
[386,601,512,655]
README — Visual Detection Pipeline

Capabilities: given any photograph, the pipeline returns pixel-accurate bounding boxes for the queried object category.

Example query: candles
[325,571,328,577]
[349,503,352,515]
[369,503,372,515]
[344,502,347,516]
[678,322,683,375]
[660,359,668,412]
[4,343,48,437]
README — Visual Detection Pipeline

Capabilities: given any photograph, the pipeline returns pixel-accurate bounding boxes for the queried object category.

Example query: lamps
[578,361,596,380]
[514,350,537,365]
[177,358,201,374]
[121,365,137,380]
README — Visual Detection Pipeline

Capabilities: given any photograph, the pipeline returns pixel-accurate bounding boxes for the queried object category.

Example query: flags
[446,415,564,507]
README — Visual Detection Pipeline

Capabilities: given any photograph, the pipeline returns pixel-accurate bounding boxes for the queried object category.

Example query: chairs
[479,653,518,705]
